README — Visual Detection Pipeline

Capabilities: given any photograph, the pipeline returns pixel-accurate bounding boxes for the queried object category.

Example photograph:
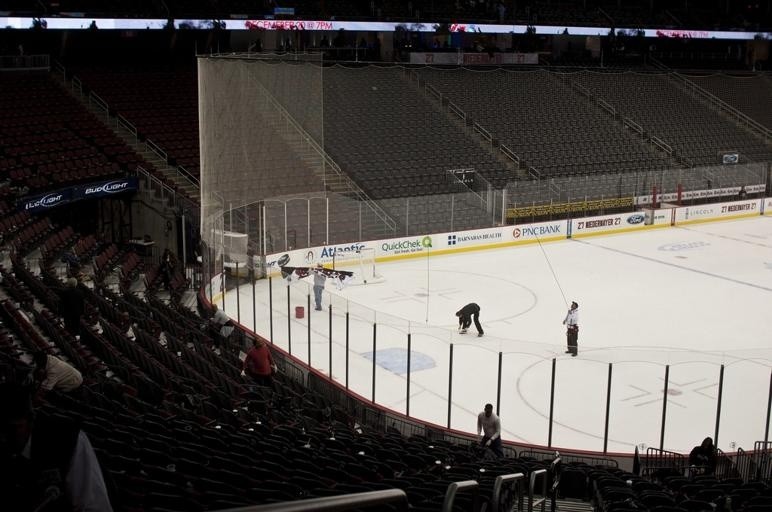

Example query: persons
[240,337,278,387]
[687,437,717,476]
[562,301,579,357]
[23,351,85,401]
[475,403,504,458]
[312,262,326,311]
[455,302,484,337]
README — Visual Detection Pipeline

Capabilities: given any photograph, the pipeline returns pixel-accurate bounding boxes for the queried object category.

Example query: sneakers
[478,332,484,336]
[565,350,577,357]
[459,330,467,334]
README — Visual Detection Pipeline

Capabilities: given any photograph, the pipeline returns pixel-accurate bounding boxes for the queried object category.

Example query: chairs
[209,64,772,290]
[2,66,378,509]
[379,416,771,511]
